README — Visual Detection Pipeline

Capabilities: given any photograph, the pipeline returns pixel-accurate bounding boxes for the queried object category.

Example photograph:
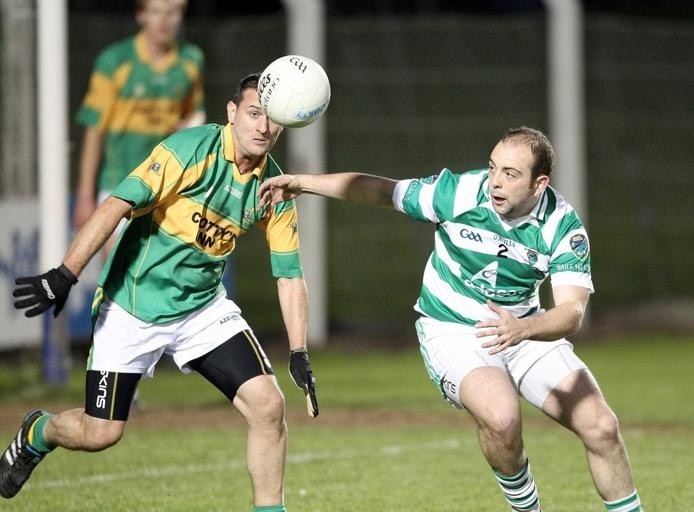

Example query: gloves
[288,348,320,419]
[12,261,79,319]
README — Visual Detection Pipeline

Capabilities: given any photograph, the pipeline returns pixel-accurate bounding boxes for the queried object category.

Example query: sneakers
[1,409,51,501]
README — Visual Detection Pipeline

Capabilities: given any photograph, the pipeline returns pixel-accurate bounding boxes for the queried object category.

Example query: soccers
[257,55,332,129]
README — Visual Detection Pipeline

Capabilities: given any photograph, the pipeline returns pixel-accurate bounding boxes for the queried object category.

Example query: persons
[254,126,644,512]
[75,0,208,411]
[1,74,320,512]
